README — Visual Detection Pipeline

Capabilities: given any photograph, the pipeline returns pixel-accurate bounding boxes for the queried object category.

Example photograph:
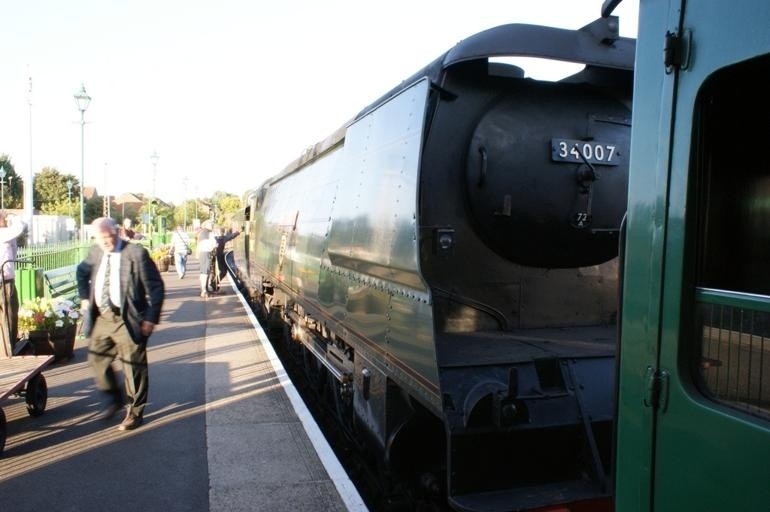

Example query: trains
[231,0,770,512]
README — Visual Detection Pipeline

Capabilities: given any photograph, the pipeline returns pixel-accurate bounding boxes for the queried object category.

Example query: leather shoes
[119,415,143,430]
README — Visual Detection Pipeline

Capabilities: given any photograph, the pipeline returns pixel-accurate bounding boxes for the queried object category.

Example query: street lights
[148,148,160,199]
[72,81,91,241]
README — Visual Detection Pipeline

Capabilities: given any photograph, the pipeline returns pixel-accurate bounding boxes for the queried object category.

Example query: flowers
[17,297,82,333]
[152,248,170,259]
[162,244,175,254]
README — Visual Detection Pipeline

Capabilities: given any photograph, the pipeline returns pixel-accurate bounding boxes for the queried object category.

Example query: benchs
[44,263,83,336]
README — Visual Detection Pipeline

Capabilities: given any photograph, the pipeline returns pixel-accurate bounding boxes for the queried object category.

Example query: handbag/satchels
[187,248,192,255]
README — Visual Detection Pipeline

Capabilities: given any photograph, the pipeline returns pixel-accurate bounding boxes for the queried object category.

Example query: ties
[100,254,115,322]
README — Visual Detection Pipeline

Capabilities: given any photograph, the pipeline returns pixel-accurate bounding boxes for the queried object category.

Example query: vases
[29,323,77,365]
[165,254,174,265]
[152,257,169,272]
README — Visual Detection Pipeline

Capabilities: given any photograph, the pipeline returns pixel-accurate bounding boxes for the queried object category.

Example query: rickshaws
[0,258,55,451]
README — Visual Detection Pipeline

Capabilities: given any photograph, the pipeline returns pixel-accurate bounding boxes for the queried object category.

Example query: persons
[121,219,147,243]
[0,208,27,346]
[76,217,164,430]
[168,224,190,279]
[196,219,245,298]
